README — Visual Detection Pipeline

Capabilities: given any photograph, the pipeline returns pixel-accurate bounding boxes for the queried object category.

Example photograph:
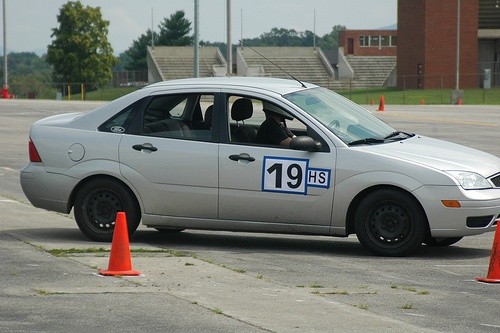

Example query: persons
[256,101,297,148]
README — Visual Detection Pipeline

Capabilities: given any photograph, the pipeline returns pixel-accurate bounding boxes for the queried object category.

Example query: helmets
[263,100,294,121]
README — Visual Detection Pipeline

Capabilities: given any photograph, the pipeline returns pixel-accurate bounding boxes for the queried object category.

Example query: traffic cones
[98,211,142,277]
[376,96,385,112]
[474,219,500,283]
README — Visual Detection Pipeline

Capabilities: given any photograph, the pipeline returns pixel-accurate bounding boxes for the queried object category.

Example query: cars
[19,74,500,257]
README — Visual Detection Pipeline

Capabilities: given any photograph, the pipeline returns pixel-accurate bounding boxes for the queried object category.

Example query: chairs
[231,97,257,143]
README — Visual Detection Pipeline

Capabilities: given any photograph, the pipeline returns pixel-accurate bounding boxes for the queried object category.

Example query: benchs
[147,117,189,136]
